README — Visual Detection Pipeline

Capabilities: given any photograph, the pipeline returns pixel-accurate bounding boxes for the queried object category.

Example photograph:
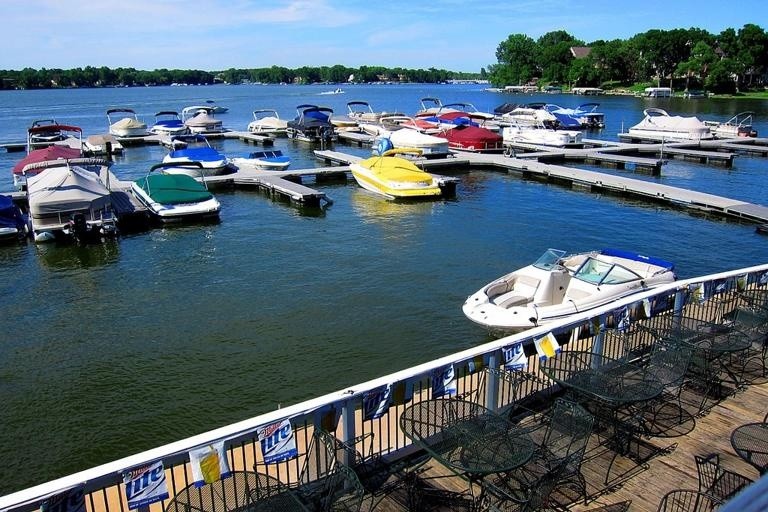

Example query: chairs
[398,286,768,511]
[245,427,477,510]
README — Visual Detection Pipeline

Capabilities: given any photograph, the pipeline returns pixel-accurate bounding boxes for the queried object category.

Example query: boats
[460,247,678,347]
[628,108,759,144]
